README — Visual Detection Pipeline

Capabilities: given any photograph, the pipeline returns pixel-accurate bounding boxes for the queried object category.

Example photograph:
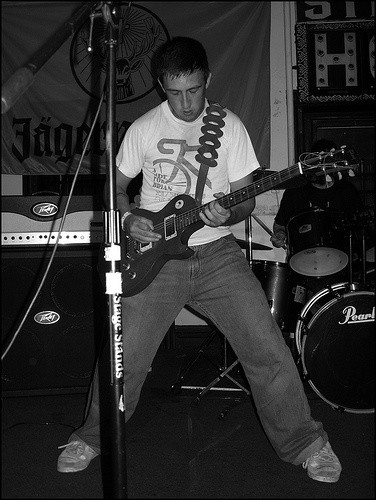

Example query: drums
[286,209,350,277]
[247,260,305,332]
[295,282,376,415]
[289,247,350,304]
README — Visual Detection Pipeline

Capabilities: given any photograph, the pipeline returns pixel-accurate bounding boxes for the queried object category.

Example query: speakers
[303,113,376,214]
[0,253,109,396]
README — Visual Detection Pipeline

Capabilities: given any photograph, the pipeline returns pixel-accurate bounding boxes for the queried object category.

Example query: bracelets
[120,211,133,232]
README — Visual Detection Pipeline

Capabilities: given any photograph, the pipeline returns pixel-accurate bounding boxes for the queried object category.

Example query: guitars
[104,145,359,298]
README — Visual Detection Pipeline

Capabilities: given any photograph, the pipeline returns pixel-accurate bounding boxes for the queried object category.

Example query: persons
[57,35,343,482]
[270,137,364,248]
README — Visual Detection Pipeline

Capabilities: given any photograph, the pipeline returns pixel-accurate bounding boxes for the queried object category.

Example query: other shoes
[56,441,100,473]
[302,441,342,483]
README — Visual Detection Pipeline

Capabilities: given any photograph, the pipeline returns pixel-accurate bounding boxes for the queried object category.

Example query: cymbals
[237,239,273,250]
